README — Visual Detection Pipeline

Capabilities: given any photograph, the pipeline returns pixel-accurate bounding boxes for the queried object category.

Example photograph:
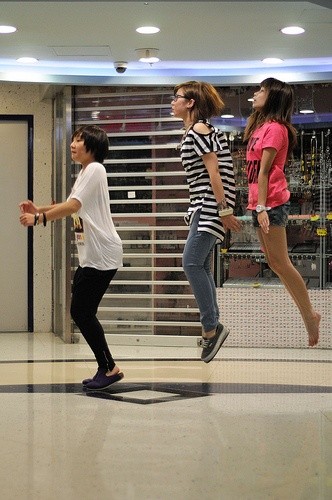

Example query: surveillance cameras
[113,61,128,74]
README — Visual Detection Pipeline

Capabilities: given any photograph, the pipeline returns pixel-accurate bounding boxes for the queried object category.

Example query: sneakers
[200,323,230,362]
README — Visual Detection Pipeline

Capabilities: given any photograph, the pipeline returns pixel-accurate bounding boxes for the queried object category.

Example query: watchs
[256,205,271,212]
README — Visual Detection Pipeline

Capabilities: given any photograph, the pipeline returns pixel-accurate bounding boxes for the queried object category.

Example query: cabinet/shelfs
[222,186,322,289]
[324,183,332,292]
[93,215,154,332]
[148,129,214,337]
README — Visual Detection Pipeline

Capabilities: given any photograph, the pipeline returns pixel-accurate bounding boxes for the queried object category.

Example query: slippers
[82,371,125,390]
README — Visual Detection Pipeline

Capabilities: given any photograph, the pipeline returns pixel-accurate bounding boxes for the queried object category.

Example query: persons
[171,81,241,362]
[18,126,123,392]
[244,78,321,347]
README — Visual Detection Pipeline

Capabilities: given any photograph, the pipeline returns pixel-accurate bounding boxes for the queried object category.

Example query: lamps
[137,49,159,64]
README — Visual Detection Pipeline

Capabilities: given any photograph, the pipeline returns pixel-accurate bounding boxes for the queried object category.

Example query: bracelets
[34,212,40,227]
[42,212,47,227]
[219,207,233,216]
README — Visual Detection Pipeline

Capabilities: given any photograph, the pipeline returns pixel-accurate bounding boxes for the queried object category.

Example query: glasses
[174,94,189,102]
